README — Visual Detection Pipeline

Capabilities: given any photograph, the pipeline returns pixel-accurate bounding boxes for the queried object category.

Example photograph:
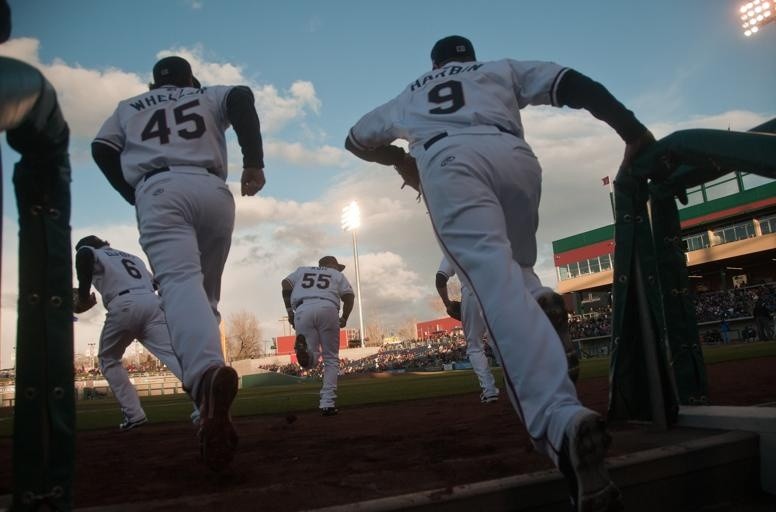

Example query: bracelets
[286,307,293,313]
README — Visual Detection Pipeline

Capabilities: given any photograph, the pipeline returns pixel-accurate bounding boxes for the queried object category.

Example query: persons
[91,56,265,467]
[345,35,657,511]
[281,256,354,417]
[76,236,184,431]
[435,253,500,403]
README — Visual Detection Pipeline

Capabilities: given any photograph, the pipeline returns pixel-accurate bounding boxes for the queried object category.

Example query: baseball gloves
[71,288,97,313]
[446,301,461,322]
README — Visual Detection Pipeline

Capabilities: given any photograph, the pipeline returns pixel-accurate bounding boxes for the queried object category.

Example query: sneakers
[119,416,148,430]
[190,410,200,423]
[199,365,239,473]
[535,292,579,384]
[295,334,309,368]
[320,407,339,416]
[480,392,500,403]
[558,410,626,511]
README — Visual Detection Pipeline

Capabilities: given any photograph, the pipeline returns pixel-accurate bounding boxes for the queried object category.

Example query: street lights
[340,199,369,347]
[84,340,96,370]
[730,0,776,40]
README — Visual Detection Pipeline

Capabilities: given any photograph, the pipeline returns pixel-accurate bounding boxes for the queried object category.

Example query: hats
[153,57,200,90]
[431,36,476,71]
[75,236,109,251]
[319,256,345,272]
